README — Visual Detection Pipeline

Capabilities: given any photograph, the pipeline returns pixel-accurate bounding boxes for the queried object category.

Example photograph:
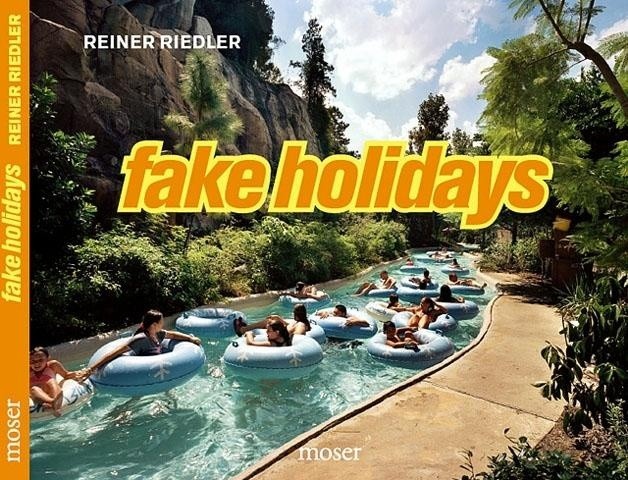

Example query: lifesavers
[366,252,485,321]
[278,290,330,307]
[222,308,457,376]
[88,333,206,395]
[29,370,95,420]
[176,307,247,338]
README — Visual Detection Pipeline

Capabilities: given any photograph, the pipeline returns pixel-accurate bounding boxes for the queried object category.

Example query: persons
[30,309,202,417]
[233,247,487,348]
